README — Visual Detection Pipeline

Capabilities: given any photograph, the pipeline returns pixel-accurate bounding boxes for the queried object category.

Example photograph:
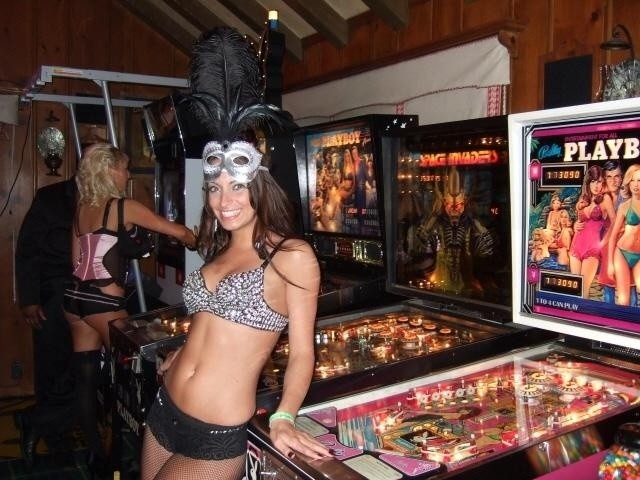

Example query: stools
[19,398,81,475]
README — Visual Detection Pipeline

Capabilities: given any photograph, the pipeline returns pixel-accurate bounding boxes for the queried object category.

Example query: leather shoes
[14,408,40,471]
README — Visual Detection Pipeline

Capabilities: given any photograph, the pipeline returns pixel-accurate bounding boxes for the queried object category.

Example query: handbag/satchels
[117,196,154,260]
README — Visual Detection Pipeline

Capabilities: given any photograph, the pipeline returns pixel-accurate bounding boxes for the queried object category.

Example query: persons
[15,134,152,468]
[529,161,640,307]
[14,142,198,479]
[142,126,337,480]
[310,145,378,233]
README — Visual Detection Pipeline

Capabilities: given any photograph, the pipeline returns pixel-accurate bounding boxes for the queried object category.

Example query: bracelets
[186,235,199,251]
[269,412,295,428]
[128,224,136,235]
